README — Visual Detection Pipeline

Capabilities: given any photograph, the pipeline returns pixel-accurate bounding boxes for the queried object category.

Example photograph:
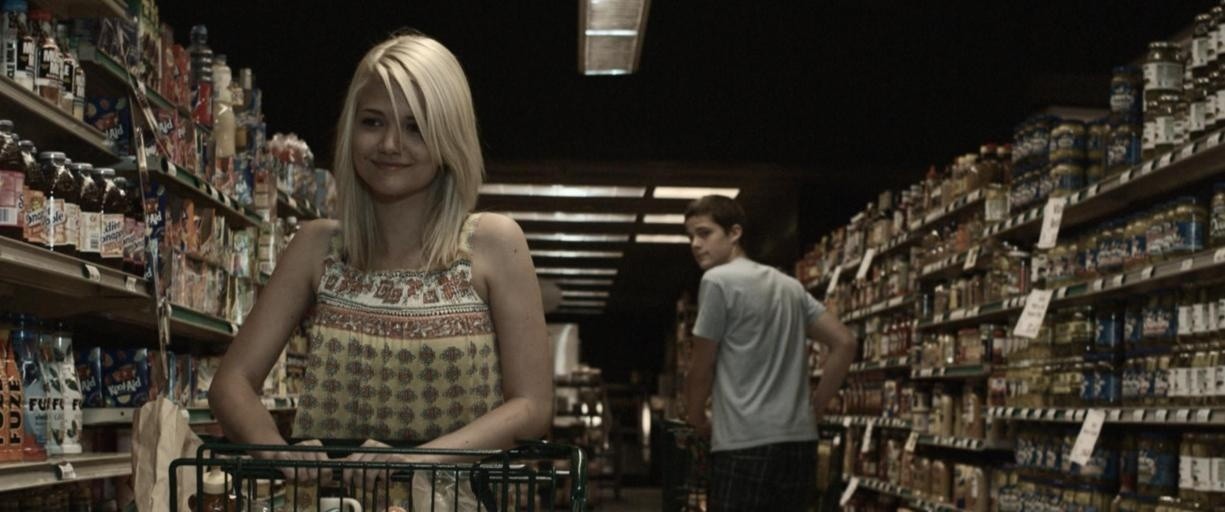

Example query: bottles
[0,113,148,279]
[0,309,85,466]
[0,0,86,123]
[187,23,235,128]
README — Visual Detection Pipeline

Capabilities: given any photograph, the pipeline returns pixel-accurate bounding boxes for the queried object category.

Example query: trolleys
[166,431,591,512]
[647,413,846,511]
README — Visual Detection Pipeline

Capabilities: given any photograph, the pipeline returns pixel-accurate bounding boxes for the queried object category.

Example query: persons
[678,195,859,510]
[203,34,557,511]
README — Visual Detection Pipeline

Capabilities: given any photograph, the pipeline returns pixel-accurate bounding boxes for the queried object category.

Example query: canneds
[910,6,1225,512]
